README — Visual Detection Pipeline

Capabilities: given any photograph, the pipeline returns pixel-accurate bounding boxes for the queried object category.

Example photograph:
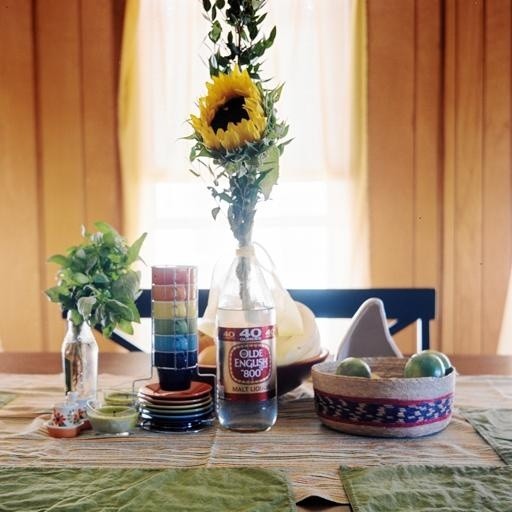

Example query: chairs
[62,289,435,352]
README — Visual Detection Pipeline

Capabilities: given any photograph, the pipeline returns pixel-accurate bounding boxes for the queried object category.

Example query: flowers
[178,0,295,324]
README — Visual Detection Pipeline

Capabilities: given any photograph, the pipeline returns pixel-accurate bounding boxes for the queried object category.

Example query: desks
[0,352,512,512]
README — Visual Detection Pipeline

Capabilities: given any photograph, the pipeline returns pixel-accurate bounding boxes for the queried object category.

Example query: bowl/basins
[103,391,140,405]
[195,351,329,402]
[44,418,84,438]
[87,406,137,432]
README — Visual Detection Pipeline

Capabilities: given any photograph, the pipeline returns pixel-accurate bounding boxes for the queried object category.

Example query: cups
[151,266,198,392]
[52,400,80,426]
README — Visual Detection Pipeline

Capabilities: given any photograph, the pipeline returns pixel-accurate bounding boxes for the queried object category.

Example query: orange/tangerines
[431,351,452,375]
[404,351,445,377]
[335,356,372,379]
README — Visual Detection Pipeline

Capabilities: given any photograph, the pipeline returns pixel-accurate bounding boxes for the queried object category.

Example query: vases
[215,248,278,432]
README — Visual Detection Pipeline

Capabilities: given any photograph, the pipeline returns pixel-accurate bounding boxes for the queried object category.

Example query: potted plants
[42,220,150,400]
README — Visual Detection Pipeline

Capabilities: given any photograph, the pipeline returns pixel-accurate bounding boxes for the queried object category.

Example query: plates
[138,381,211,428]
